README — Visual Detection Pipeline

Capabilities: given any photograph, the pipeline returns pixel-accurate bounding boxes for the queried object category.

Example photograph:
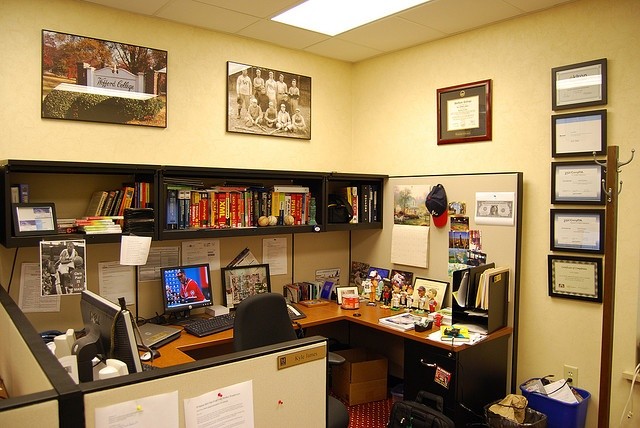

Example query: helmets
[176,269,186,277]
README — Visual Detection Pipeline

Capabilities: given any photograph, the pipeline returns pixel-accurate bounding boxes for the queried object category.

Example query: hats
[425,184,447,227]
[280,103,286,108]
[251,99,257,103]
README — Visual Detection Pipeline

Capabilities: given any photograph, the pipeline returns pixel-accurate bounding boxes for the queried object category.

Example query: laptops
[134,322,182,348]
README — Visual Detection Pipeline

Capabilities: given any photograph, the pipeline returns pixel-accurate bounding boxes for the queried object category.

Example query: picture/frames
[551,109,608,157]
[409,277,450,312]
[551,160,606,205]
[551,57,607,110]
[549,208,605,254]
[225,60,312,140]
[221,263,272,312]
[9,200,58,239]
[40,28,168,129]
[547,254,603,303]
[436,79,492,144]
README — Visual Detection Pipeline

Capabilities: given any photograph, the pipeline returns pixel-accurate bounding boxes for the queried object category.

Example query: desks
[132,294,513,427]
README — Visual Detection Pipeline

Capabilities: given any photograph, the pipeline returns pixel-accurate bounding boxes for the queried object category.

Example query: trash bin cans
[520,377,591,428]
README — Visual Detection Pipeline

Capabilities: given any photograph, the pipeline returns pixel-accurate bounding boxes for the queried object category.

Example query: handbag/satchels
[390,400,455,428]
[328,194,353,223]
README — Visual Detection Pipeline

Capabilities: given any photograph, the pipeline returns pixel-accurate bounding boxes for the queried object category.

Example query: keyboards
[184,311,236,338]
[141,362,161,372]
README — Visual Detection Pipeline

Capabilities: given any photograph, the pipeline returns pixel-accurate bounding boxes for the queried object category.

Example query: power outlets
[563,365,579,387]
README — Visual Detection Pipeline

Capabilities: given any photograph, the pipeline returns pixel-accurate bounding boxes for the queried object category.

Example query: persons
[256,86,270,112]
[414,286,427,314]
[244,98,264,127]
[264,71,277,109]
[352,268,367,286]
[390,270,407,286]
[276,73,287,95]
[289,108,307,133]
[288,79,301,113]
[426,288,438,314]
[175,269,206,304]
[236,69,253,119]
[372,271,382,283]
[264,102,279,127]
[57,241,79,294]
[275,104,292,131]
[253,69,265,96]
[277,94,291,115]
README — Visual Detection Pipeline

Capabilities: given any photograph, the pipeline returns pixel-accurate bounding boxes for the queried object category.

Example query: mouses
[140,349,160,362]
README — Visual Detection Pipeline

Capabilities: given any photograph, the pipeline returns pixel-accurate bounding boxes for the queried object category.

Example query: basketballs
[73,256,83,266]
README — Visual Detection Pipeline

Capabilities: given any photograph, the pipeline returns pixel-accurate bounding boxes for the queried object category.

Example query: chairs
[233,294,298,353]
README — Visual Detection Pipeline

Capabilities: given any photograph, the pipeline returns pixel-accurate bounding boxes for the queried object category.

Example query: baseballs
[258,216,268,226]
[284,215,294,225]
[268,216,278,226]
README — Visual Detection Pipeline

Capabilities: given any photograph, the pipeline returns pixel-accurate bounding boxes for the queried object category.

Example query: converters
[450,328,460,336]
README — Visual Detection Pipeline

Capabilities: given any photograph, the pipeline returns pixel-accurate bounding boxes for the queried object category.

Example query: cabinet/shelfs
[3,159,385,249]
[403,336,509,427]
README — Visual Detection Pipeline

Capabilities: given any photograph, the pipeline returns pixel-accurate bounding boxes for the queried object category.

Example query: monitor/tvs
[71,289,143,384]
[159,263,214,320]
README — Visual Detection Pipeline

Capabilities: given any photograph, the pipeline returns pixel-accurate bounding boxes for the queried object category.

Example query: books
[319,281,335,301]
[57,182,152,234]
[13,182,29,204]
[11,186,20,204]
[338,183,379,224]
[283,280,323,304]
[439,325,471,343]
[427,306,452,325]
[163,176,319,230]
[378,312,435,332]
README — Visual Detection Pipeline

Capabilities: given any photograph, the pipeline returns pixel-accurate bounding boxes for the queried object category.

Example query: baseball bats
[257,124,267,133]
[268,128,281,134]
[235,126,257,133]
